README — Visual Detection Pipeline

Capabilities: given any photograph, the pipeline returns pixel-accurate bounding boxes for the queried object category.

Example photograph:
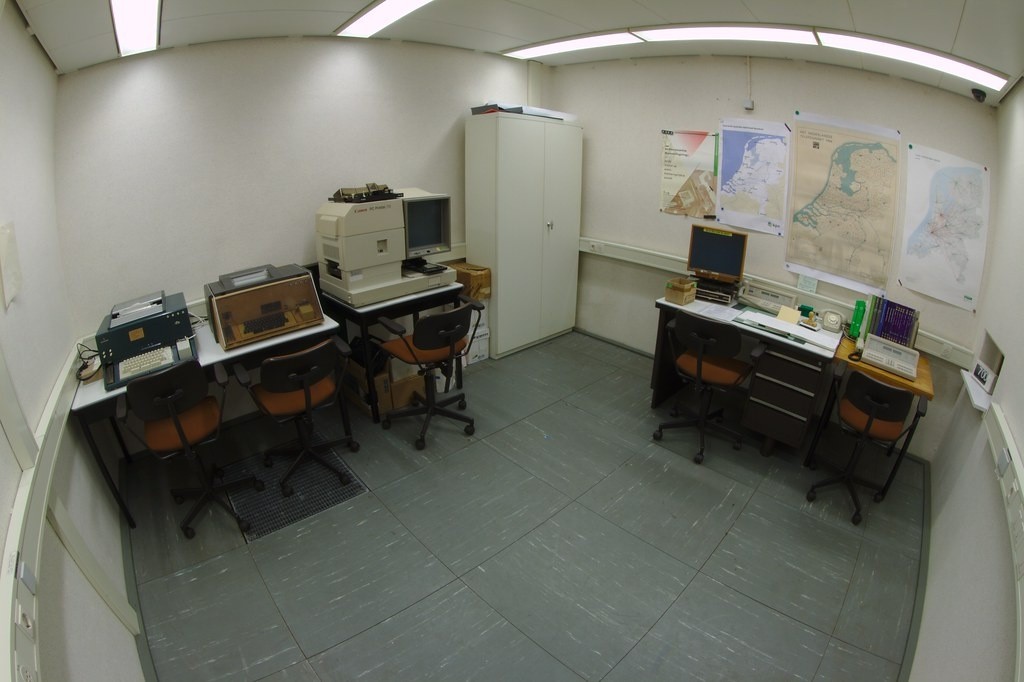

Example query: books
[849,293,921,347]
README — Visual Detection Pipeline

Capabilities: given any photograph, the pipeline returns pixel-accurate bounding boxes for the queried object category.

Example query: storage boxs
[340,261,492,415]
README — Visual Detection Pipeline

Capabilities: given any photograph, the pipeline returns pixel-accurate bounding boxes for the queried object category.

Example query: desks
[193,314,349,440]
[325,283,464,426]
[74,349,207,529]
[652,294,936,453]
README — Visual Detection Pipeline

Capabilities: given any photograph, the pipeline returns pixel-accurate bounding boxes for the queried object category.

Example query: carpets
[215,430,367,543]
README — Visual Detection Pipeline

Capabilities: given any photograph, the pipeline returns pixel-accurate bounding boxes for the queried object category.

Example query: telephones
[819,309,846,333]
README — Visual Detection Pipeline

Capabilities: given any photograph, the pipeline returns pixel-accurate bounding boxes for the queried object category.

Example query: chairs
[366,293,479,449]
[123,359,264,536]
[233,329,361,495]
[806,361,931,524]
[653,310,767,464]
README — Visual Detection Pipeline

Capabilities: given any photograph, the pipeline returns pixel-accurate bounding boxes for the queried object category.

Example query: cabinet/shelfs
[465,113,583,359]
